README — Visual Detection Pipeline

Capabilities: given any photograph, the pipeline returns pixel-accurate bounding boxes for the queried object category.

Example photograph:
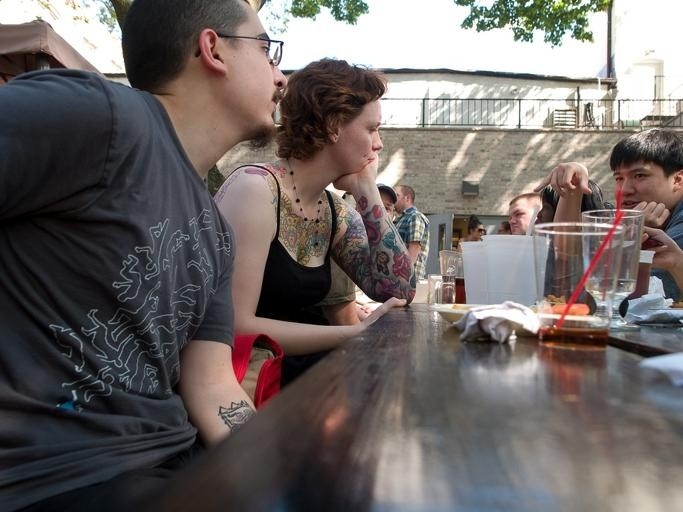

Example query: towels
[624,294,683,323]
[451,300,542,344]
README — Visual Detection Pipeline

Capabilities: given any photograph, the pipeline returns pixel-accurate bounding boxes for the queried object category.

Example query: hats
[376,183,397,204]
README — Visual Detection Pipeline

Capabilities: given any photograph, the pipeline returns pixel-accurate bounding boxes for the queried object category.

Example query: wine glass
[580,209,646,332]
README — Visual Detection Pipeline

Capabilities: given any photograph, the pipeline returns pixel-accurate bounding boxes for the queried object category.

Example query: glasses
[475,226,486,233]
[194,32,284,67]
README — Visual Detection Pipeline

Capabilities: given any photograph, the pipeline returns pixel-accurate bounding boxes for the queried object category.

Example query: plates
[430,303,600,336]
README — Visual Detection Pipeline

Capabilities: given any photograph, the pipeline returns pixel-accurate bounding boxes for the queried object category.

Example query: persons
[213,56,417,386]
[392,184,431,283]
[375,183,399,218]
[316,193,373,325]
[0,0,294,510]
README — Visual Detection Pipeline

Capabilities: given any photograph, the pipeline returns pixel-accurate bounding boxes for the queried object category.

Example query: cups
[532,222,625,354]
[426,233,551,305]
[619,250,655,316]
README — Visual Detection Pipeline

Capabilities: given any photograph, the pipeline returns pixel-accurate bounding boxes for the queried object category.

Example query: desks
[640,114,677,126]
